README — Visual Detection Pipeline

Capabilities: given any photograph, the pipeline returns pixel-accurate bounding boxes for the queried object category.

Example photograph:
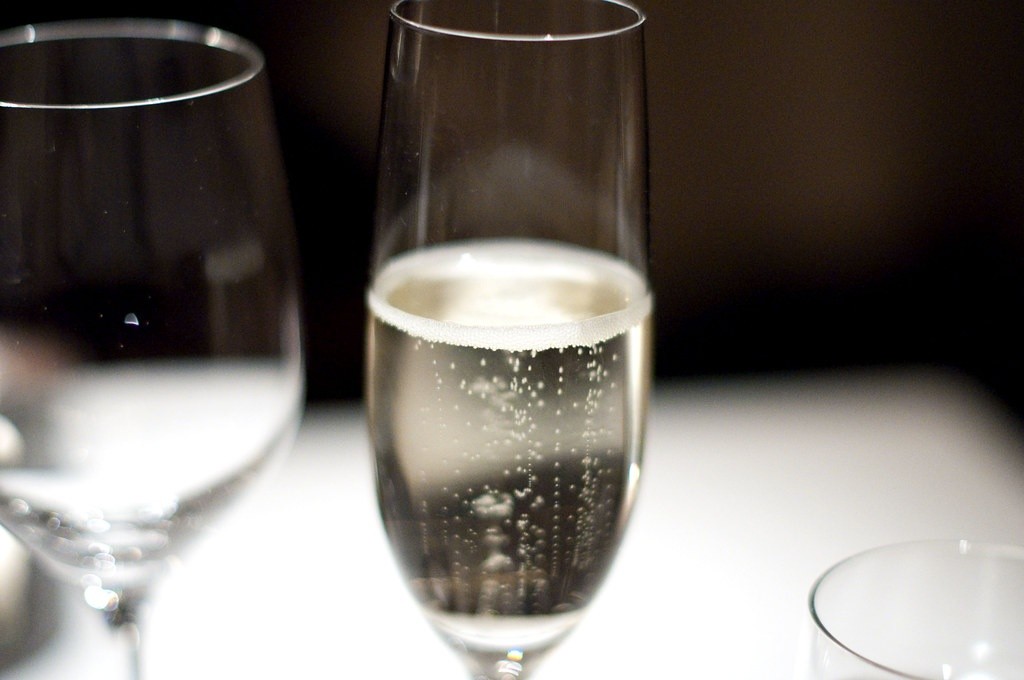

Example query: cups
[810,538,1024,680]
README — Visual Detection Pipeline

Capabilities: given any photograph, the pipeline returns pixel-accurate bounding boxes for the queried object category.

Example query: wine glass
[368,0,653,680]
[0,26,308,680]
